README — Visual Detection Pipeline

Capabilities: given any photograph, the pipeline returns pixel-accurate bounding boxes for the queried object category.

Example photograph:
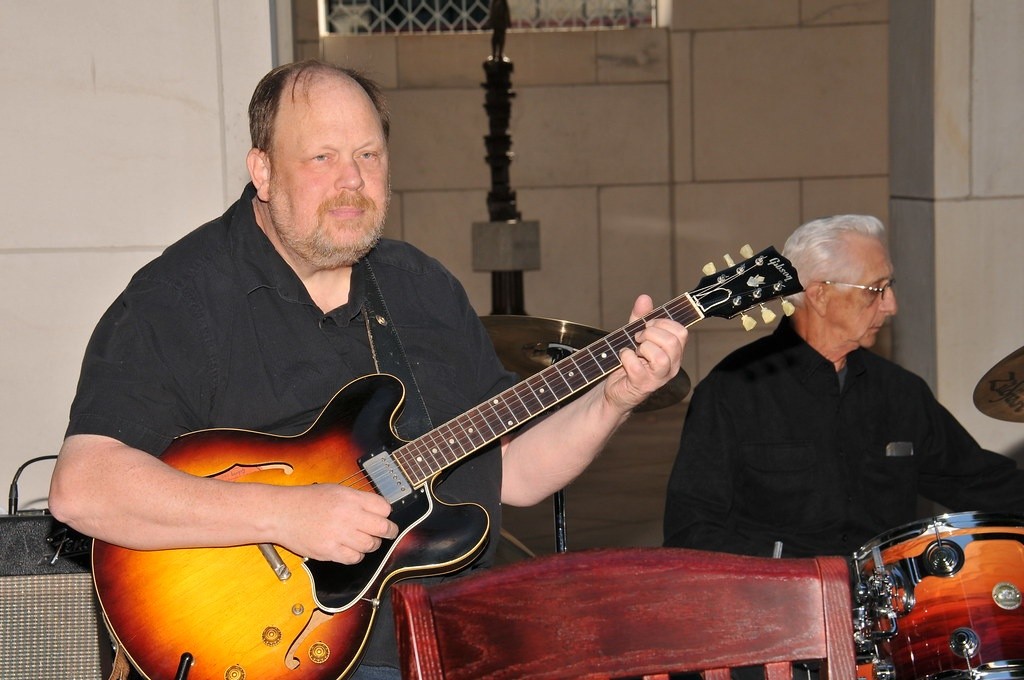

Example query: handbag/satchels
[0,455,93,576]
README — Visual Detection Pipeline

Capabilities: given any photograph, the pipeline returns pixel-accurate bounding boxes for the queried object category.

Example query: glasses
[803,278,898,301]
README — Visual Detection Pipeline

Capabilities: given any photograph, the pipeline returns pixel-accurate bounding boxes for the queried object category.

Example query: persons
[664,214,1023,556]
[48,57,689,680]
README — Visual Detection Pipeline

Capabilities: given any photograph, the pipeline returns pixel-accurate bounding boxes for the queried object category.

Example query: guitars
[85,243,812,679]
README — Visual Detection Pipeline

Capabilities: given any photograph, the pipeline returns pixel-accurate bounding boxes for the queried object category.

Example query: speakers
[0,508,116,680]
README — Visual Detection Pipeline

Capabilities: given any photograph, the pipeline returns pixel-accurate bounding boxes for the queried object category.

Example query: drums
[848,510,1024,680]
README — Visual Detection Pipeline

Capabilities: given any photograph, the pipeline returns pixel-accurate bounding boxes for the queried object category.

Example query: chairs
[389,547,856,680]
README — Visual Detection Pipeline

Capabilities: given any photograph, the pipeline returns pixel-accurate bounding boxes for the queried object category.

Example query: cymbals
[972,347,1024,426]
[476,314,694,415]
